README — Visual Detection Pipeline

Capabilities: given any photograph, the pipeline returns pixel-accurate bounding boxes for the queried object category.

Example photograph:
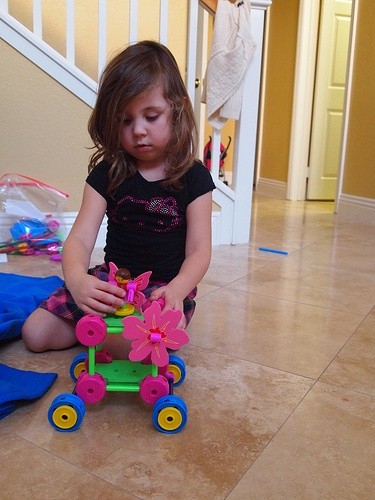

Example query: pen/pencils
[258,248,288,255]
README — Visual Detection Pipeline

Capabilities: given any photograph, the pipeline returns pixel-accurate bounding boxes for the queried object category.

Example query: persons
[20,39,220,365]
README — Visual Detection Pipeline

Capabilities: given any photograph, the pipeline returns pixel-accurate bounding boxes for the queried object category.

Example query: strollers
[202,134,232,186]
